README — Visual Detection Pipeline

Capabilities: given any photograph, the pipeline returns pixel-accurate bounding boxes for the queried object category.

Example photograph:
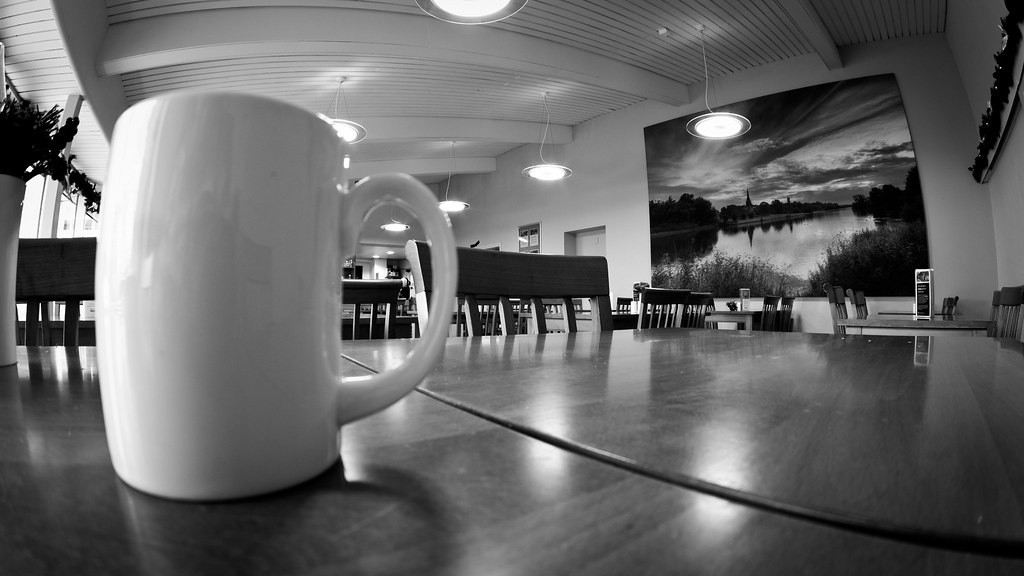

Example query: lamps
[522,93,574,181]
[319,76,366,146]
[436,140,471,213]
[684,25,751,140]
[414,0,530,25]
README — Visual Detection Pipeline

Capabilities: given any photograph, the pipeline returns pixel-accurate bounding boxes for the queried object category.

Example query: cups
[742,299,749,311]
[97,91,459,501]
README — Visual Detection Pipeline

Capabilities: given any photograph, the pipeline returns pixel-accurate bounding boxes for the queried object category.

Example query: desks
[519,311,711,334]
[0,334,1024,576]
[343,313,416,325]
[706,310,786,336]
[839,317,998,333]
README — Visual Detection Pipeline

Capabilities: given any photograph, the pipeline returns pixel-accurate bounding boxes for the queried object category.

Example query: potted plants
[0,93,99,367]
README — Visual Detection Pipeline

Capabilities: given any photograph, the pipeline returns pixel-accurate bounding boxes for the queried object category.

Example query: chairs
[636,288,691,331]
[342,239,634,340]
[781,297,795,332]
[822,282,1024,340]
[681,292,713,328]
[760,294,780,332]
